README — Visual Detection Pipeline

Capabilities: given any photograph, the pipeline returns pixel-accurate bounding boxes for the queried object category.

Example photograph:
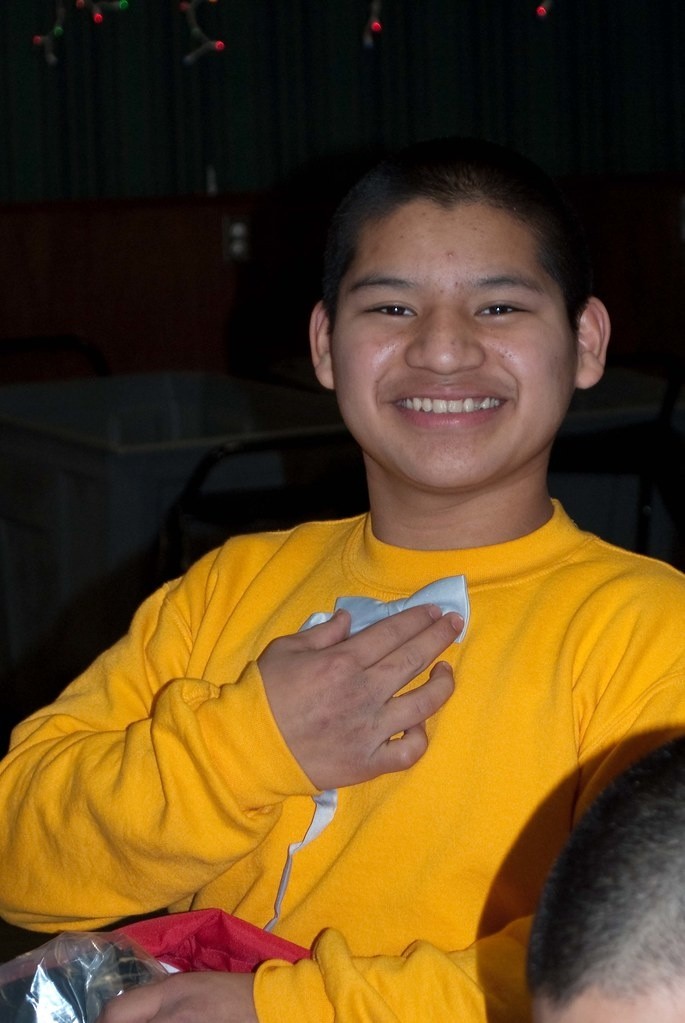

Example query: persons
[0,137,685,1023]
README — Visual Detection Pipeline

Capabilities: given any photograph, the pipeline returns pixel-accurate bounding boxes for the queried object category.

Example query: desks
[0,372,667,756]
